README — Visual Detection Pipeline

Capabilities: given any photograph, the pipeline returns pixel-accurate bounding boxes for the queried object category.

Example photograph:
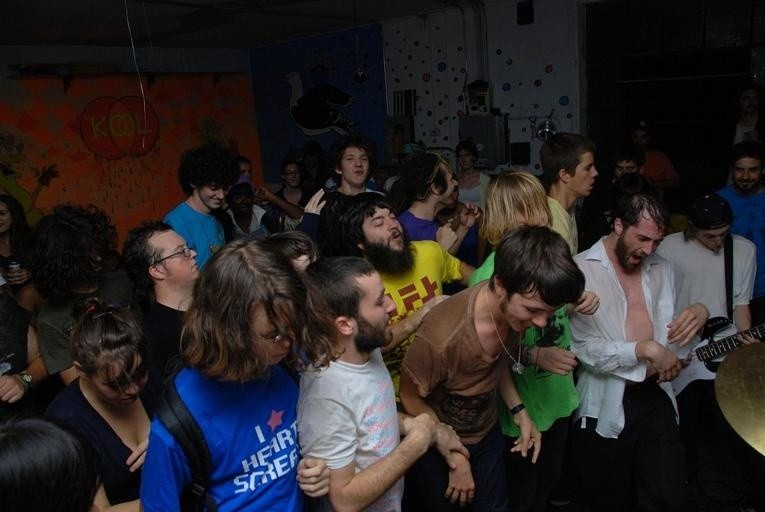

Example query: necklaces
[190,195,218,243]
[486,281,524,375]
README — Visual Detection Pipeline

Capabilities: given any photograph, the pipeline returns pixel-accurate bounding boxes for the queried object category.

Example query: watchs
[511,403,525,415]
[15,372,32,389]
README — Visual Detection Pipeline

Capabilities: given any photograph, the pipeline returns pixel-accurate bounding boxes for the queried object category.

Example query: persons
[0,113,765,511]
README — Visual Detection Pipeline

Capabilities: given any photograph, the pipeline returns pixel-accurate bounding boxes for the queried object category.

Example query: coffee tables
[715,342,765,457]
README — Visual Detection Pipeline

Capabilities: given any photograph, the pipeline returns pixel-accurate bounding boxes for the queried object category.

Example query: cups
[153,246,196,264]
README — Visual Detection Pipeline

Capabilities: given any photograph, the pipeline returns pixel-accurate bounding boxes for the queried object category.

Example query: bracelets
[534,346,542,363]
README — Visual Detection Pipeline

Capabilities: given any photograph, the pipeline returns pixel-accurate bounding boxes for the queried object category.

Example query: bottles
[686,192,733,231]
